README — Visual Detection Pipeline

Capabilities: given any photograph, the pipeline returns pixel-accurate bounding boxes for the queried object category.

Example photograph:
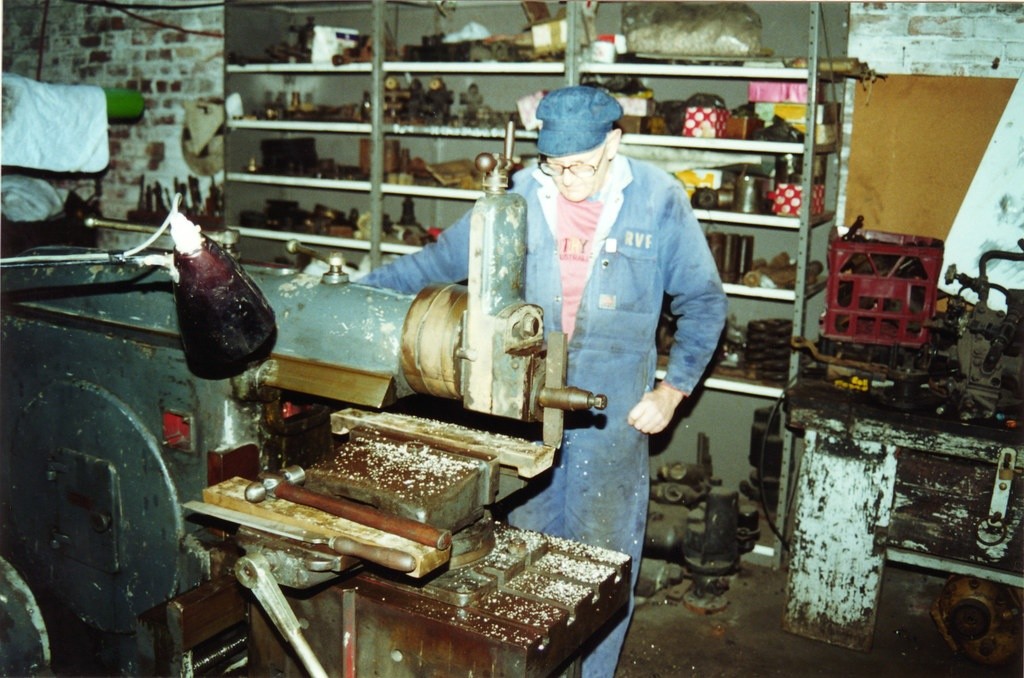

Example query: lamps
[0,193,279,382]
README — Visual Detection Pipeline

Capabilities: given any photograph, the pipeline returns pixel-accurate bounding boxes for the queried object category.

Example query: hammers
[243,462,452,552]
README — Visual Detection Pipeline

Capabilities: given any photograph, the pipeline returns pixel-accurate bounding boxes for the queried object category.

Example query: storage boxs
[824,223,943,348]
[785,378,1024,593]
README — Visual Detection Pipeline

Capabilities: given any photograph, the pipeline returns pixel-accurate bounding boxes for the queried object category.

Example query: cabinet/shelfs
[221,0,822,574]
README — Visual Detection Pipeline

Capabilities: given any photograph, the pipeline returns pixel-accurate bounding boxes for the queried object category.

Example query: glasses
[536,141,609,178]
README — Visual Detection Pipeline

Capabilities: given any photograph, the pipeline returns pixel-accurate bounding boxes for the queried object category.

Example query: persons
[348,87,728,678]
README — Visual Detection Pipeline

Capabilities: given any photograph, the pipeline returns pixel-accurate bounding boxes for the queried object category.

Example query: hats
[535,86,623,157]
